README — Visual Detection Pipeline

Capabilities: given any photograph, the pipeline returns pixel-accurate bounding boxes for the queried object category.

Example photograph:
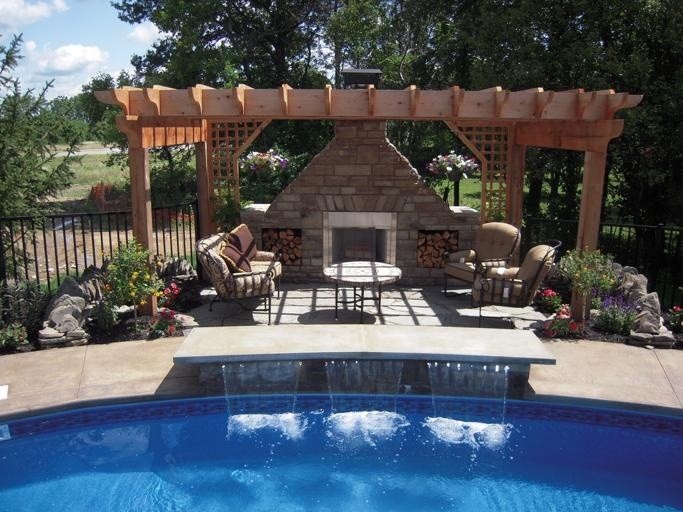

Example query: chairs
[444,222,522,307]
[472,239,562,328]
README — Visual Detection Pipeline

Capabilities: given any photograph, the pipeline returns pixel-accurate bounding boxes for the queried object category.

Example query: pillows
[219,243,251,272]
[225,223,257,261]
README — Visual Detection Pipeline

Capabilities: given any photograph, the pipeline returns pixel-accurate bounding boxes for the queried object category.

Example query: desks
[323,261,402,324]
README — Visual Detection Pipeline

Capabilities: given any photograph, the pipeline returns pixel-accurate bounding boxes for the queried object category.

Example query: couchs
[196,233,282,325]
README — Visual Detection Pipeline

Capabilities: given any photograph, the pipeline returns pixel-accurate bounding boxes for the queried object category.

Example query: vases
[448,173,461,181]
[255,175,274,183]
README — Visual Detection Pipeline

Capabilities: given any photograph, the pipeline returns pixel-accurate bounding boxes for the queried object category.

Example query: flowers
[425,150,480,179]
[241,149,288,177]
